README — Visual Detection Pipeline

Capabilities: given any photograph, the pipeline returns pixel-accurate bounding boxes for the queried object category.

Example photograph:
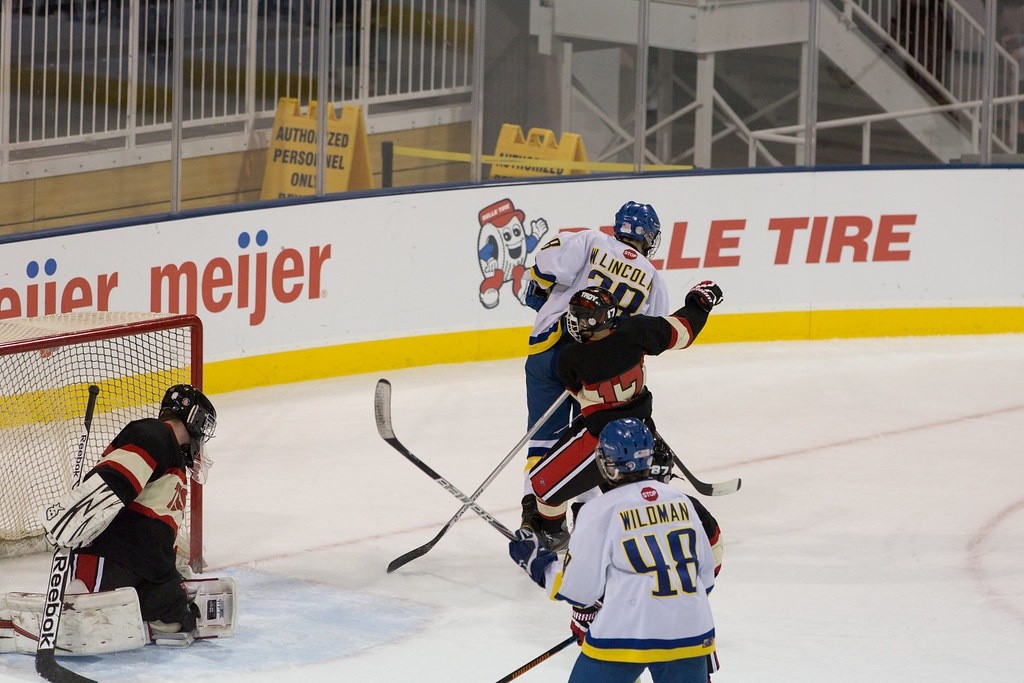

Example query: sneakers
[519,494,571,552]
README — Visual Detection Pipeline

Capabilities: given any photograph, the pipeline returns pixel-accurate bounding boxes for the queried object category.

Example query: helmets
[596,417,654,481]
[159,383,217,468]
[613,201,661,260]
[565,284,618,342]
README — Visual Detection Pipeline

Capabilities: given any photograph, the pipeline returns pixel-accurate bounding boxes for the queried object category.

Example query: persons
[524,201,670,579]
[529,281,724,576]
[509,417,719,683]
[0,384,237,657]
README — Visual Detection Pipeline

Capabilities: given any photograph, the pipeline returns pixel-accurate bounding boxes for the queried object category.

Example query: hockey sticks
[653,430,742,496]
[494,632,580,683]
[385,386,572,575]
[33,384,101,683]
[373,378,605,612]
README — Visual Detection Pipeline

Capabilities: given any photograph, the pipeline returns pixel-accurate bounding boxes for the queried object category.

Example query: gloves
[685,280,724,313]
[569,605,599,647]
[525,279,550,312]
[508,528,558,589]
